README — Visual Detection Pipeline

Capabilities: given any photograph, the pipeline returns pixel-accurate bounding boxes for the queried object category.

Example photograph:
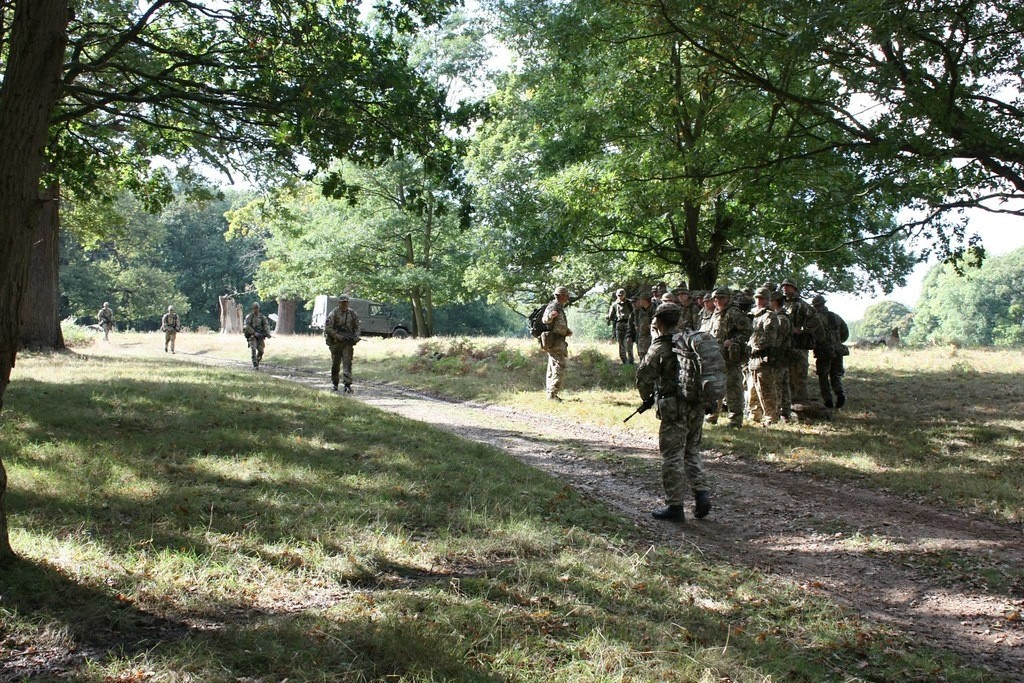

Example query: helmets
[168,305,175,311]
[652,303,681,318]
[338,294,350,302]
[252,302,260,308]
[616,278,828,307]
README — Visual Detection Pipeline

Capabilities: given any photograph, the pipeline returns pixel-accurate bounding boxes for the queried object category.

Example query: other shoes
[332,382,338,391]
[165,347,175,354]
[254,366,258,370]
[546,378,846,430]
[344,385,354,394]
[695,498,712,518]
[652,505,686,523]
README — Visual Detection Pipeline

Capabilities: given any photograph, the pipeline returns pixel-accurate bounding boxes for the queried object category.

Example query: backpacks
[651,331,728,406]
[529,304,551,337]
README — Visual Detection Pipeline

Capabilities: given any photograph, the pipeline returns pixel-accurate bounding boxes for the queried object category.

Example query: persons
[635,301,711,520]
[608,277,848,428]
[162,305,180,354]
[541,285,572,404]
[98,301,112,341]
[244,301,271,370]
[325,293,360,393]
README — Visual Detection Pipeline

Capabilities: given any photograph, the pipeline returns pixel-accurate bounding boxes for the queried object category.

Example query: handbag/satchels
[730,344,752,364]
[824,344,849,359]
[796,334,815,349]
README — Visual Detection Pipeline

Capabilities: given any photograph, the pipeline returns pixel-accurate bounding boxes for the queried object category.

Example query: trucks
[308,294,413,339]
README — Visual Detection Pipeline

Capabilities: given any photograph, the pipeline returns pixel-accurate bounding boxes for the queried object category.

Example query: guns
[169,324,183,334]
[338,331,367,344]
[254,327,276,339]
[623,394,655,423]
[104,316,115,327]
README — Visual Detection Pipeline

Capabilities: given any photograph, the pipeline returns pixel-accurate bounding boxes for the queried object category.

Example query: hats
[553,286,571,294]
